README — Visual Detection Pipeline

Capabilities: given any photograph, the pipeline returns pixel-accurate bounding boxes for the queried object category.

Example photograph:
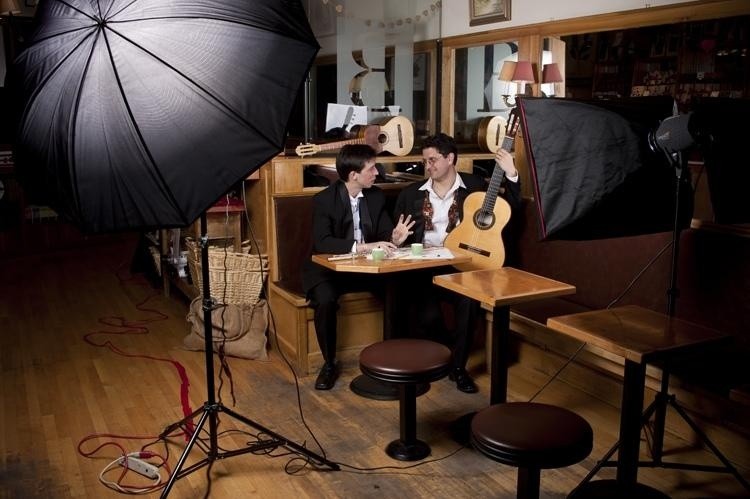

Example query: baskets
[185,236,270,304]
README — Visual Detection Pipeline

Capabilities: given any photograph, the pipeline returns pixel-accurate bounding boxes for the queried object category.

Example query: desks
[545,304,720,499]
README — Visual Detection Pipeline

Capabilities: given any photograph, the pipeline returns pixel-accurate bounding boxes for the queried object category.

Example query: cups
[372,249,384,263]
[411,243,424,256]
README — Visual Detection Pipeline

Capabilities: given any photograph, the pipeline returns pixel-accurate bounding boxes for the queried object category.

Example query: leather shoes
[315,360,340,390]
[449,367,479,393]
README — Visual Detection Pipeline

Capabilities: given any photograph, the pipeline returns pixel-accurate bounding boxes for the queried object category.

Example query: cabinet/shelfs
[161,194,248,303]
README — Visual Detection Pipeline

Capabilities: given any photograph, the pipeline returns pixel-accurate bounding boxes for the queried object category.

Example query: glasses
[423,155,444,167]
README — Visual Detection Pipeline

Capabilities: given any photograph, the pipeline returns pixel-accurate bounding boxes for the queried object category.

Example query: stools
[359,338,454,462]
[470,401,594,498]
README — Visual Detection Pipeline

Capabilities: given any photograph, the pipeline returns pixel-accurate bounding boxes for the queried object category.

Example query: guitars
[443,107,521,270]
[295,115,415,159]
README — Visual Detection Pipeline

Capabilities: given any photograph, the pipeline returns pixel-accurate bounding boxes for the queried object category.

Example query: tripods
[564,166,749,499]
[142,212,342,499]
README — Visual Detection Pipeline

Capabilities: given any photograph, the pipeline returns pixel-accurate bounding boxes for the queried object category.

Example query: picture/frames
[468,0,513,28]
[389,53,428,93]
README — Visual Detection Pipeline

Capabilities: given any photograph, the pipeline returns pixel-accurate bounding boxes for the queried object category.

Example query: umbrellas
[0,1,324,241]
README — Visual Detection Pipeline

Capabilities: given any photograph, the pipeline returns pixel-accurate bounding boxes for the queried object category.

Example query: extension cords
[118,451,159,480]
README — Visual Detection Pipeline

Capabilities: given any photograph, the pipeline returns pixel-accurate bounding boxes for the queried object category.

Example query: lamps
[541,62,563,99]
[497,60,536,108]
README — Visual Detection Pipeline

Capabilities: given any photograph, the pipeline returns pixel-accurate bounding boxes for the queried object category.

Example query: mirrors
[442,27,518,154]
[538,1,750,99]
[309,39,436,141]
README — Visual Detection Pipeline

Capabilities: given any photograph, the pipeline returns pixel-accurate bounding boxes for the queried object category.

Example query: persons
[302,143,416,391]
[393,131,527,394]
